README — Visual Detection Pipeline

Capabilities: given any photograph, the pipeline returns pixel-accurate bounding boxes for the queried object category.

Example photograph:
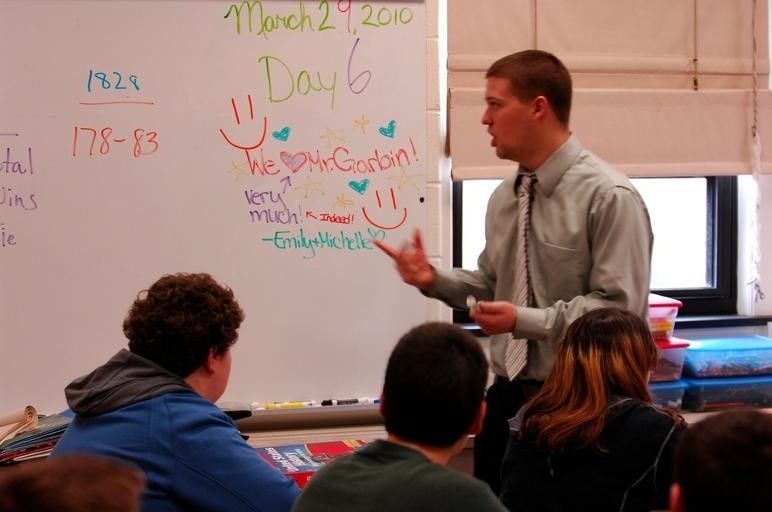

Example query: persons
[52,270,302,512]
[668,409,772,512]
[371,51,654,498]
[281,322,509,512]
[502,306,682,512]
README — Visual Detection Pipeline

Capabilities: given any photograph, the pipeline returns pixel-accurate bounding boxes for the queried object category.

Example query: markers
[322,398,380,405]
[266,400,315,408]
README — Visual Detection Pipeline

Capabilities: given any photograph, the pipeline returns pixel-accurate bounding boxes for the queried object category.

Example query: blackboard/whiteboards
[0,0,453,431]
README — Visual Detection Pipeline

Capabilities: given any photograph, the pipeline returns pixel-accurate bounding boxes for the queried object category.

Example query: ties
[503,171,536,381]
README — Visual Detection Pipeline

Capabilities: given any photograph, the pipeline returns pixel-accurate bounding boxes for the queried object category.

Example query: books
[2,407,72,464]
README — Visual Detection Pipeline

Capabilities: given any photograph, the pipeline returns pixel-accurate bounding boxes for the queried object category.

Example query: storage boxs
[645,291,772,411]
